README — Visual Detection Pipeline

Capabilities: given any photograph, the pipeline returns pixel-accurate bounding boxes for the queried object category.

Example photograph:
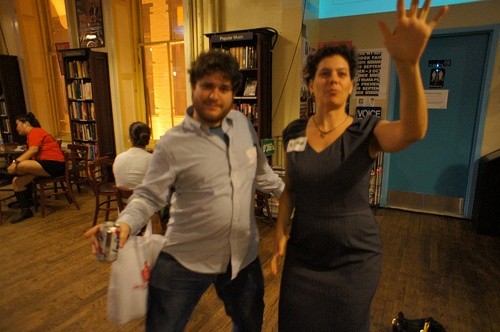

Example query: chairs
[0,139,148,253]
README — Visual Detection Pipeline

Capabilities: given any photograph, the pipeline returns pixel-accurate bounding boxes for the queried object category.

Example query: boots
[12,188,34,222]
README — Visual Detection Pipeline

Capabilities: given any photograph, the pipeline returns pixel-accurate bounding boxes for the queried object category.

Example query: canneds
[97,221,120,263]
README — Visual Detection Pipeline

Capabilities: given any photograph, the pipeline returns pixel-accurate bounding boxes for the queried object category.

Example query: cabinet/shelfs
[58,47,118,184]
[0,54,27,160]
[202,28,276,220]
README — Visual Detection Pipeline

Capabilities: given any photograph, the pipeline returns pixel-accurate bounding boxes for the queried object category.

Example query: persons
[4,112,64,224]
[82,49,285,332]
[269,0,453,332]
[112,121,175,225]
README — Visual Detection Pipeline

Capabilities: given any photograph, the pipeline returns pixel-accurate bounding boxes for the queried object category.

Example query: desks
[0,143,51,209]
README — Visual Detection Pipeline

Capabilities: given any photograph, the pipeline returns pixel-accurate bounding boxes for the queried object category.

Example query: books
[63,56,104,182]
[214,44,266,129]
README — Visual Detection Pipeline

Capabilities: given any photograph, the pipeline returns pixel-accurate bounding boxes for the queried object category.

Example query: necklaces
[311,114,351,137]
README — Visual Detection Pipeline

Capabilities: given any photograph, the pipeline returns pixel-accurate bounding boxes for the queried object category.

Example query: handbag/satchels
[107,198,167,322]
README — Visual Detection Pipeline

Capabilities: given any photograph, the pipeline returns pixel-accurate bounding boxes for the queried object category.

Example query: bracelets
[13,159,19,163]
